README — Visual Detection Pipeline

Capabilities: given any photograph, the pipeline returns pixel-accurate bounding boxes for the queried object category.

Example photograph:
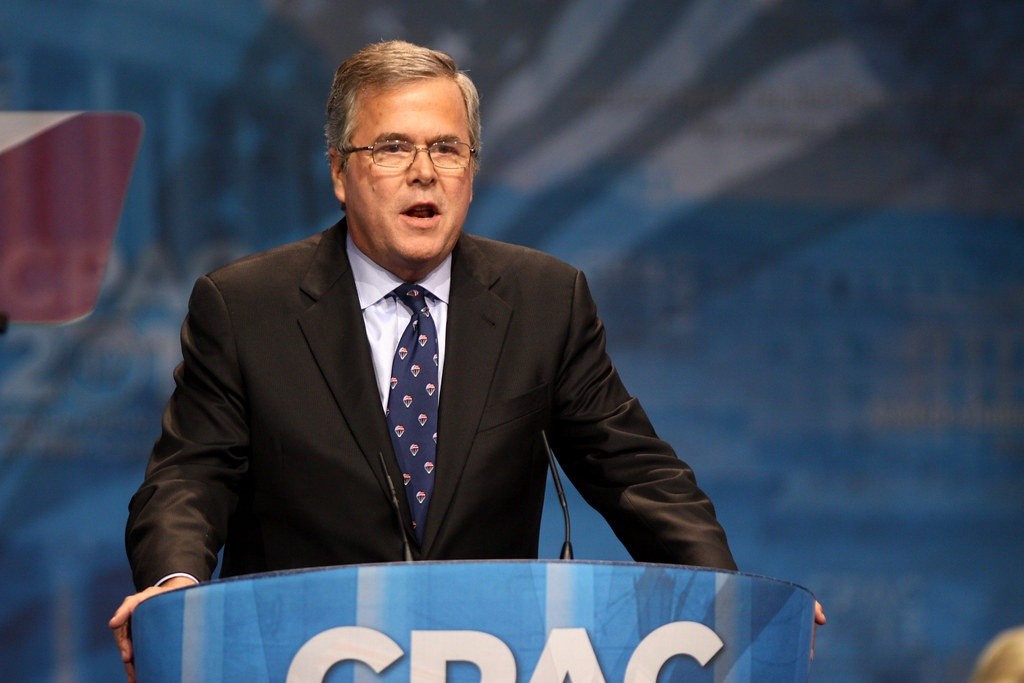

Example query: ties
[385,283,439,553]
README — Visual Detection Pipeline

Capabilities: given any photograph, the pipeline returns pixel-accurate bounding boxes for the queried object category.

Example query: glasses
[343,139,476,169]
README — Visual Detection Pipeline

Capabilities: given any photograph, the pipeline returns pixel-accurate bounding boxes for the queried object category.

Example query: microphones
[378,451,413,562]
[541,429,574,560]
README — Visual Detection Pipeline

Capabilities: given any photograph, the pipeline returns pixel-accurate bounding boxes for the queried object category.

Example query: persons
[109,39,825,683]
